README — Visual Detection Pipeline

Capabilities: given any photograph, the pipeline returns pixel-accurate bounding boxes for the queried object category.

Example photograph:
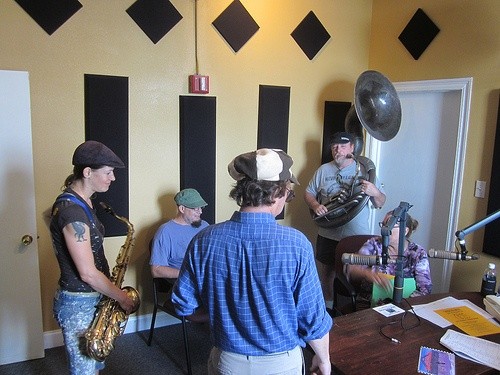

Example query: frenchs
[308,70,402,228]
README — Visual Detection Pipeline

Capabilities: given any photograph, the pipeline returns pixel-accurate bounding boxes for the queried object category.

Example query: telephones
[483,295,500,321]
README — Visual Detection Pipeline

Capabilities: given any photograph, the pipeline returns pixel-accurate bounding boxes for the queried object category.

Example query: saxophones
[86,195,140,362]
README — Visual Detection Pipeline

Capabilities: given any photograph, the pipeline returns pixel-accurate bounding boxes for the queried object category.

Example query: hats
[328,132,354,143]
[228,148,301,185]
[72,140,126,168]
[174,188,209,208]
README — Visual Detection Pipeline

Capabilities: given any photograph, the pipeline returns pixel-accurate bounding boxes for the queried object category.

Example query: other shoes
[326,308,334,318]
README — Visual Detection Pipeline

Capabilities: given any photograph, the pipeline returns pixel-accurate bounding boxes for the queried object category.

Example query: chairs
[146,237,211,375]
[331,235,381,317]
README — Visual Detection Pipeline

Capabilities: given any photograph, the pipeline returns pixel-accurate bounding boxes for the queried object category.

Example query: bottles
[481,262,496,297]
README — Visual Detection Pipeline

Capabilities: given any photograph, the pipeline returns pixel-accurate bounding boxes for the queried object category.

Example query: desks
[304,291,500,375]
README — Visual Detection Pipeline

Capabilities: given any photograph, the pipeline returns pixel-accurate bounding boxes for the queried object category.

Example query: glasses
[188,207,204,211]
[285,187,296,202]
[379,222,401,228]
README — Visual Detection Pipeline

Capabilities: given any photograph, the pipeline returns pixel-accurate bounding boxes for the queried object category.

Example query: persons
[304,132,386,303]
[41,141,132,375]
[171,148,333,375]
[149,188,210,285]
[348,209,433,311]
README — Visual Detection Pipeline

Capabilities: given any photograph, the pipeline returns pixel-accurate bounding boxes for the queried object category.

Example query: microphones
[341,253,407,266]
[428,248,478,261]
